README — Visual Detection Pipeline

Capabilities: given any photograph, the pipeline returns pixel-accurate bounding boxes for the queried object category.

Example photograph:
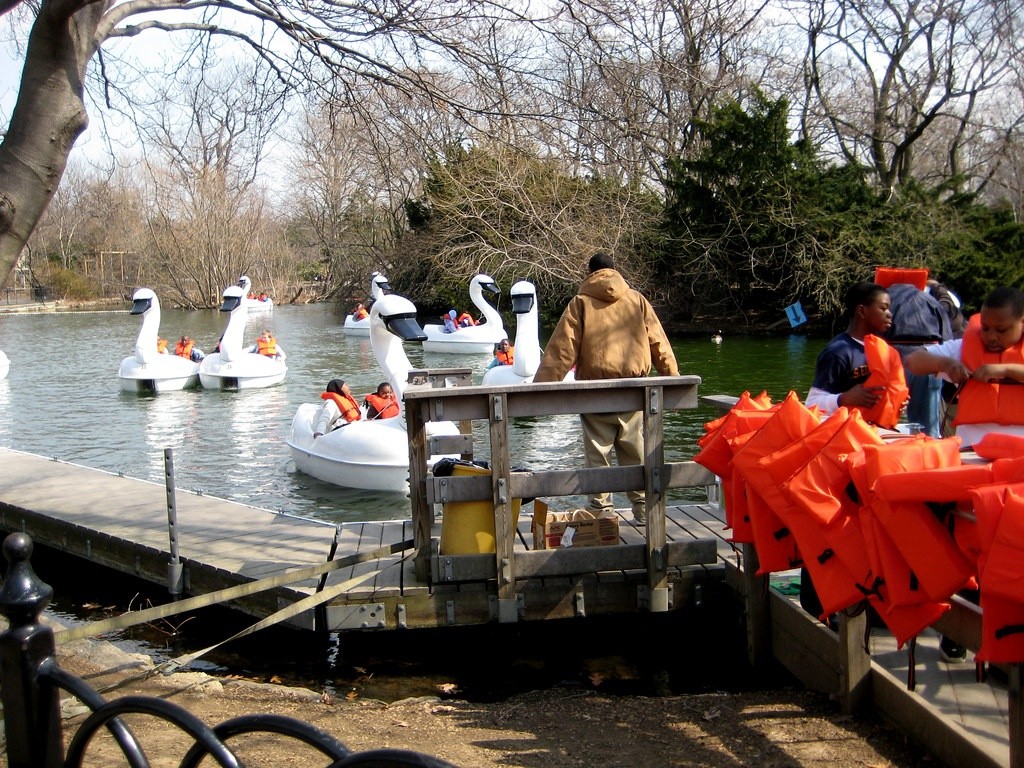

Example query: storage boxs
[532,499,619,550]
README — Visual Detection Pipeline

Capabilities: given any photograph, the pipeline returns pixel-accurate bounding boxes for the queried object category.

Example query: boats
[198,286,288,390]
[285,294,459,492]
[237,275,274,309]
[481,281,576,386]
[342,276,391,336]
[118,288,199,392]
[421,274,508,354]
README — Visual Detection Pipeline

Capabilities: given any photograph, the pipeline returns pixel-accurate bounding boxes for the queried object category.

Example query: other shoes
[939,634,966,662]
[634,514,646,525]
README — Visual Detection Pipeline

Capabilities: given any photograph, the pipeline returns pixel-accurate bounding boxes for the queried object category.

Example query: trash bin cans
[440,459,522,555]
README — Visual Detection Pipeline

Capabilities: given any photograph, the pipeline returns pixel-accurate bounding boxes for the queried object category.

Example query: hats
[327,379,346,398]
[589,253,613,272]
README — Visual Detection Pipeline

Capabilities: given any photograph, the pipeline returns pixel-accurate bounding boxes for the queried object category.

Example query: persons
[906,287,1024,662]
[251,329,276,360]
[351,302,369,322]
[799,282,910,634]
[440,309,486,334]
[247,291,268,303]
[157,335,226,360]
[315,380,399,439]
[885,279,968,516]
[533,254,681,527]
[484,339,515,375]
